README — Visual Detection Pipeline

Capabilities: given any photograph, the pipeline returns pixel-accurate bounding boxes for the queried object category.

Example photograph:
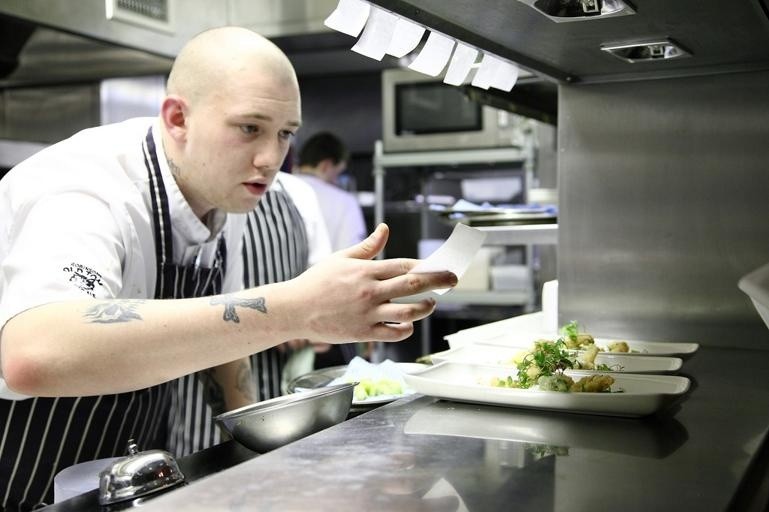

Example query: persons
[276,129,371,371]
[0,26,460,512]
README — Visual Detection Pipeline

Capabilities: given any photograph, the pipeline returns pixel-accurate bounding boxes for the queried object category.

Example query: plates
[406,327,699,417]
[525,186,559,208]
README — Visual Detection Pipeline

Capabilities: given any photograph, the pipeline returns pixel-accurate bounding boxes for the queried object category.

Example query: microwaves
[380,66,528,150]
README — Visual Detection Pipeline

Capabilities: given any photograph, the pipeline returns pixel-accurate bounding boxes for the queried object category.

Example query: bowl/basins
[211,382,359,454]
[288,362,437,408]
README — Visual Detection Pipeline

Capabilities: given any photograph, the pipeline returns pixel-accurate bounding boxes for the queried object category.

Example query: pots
[421,177,462,196]
